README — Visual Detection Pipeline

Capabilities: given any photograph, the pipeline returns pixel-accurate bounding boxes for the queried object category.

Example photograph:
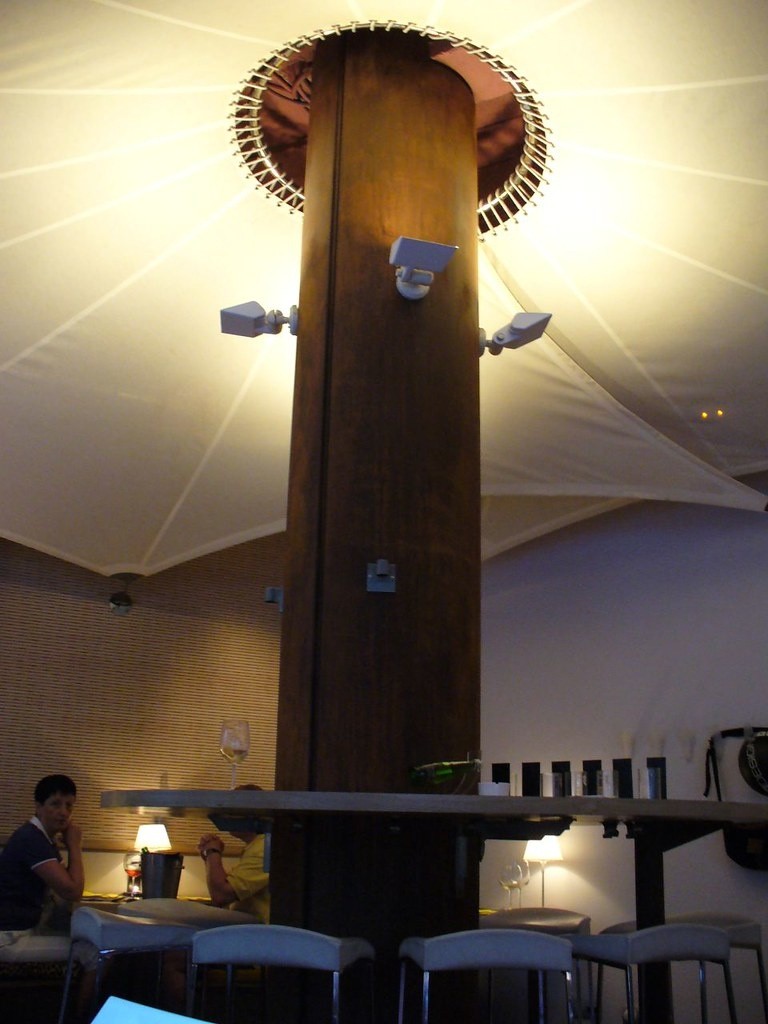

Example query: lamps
[134,824,172,855]
[263,585,284,614]
[478,311,554,358]
[365,558,398,592]
[522,832,564,908]
[388,234,460,301]
[220,300,299,338]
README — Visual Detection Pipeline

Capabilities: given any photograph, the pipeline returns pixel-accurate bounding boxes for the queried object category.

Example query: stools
[0,903,768,1024]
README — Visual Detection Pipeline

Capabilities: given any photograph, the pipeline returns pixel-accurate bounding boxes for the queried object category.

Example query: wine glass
[220,719,251,790]
[510,860,531,908]
[123,853,142,903]
[497,864,525,910]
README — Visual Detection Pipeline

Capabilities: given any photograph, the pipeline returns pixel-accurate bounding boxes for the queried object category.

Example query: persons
[0,774,101,1001]
[199,784,270,925]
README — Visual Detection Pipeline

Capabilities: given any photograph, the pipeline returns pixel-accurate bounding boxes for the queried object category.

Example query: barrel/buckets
[141,853,183,899]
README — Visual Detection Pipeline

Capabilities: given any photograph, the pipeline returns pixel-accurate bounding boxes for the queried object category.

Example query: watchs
[201,848,221,860]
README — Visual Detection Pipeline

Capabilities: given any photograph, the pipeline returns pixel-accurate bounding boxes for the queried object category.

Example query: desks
[99,789,768,1024]
